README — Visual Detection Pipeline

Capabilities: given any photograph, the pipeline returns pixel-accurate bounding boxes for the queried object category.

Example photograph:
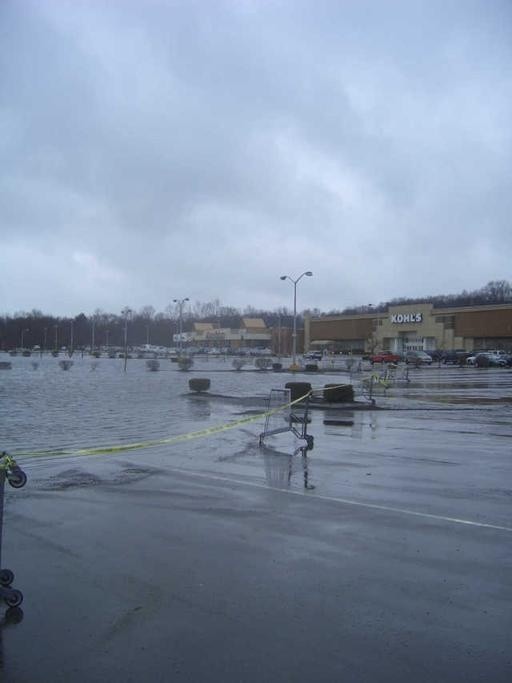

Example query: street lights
[173,298,189,353]
[368,304,379,327]
[44,320,73,352]
[280,271,312,365]
[21,328,29,348]
[121,309,132,371]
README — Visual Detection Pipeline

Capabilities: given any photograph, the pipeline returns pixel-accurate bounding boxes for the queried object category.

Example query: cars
[140,344,273,357]
[303,350,323,361]
[404,350,512,367]
[362,350,400,365]
[31,345,41,351]
